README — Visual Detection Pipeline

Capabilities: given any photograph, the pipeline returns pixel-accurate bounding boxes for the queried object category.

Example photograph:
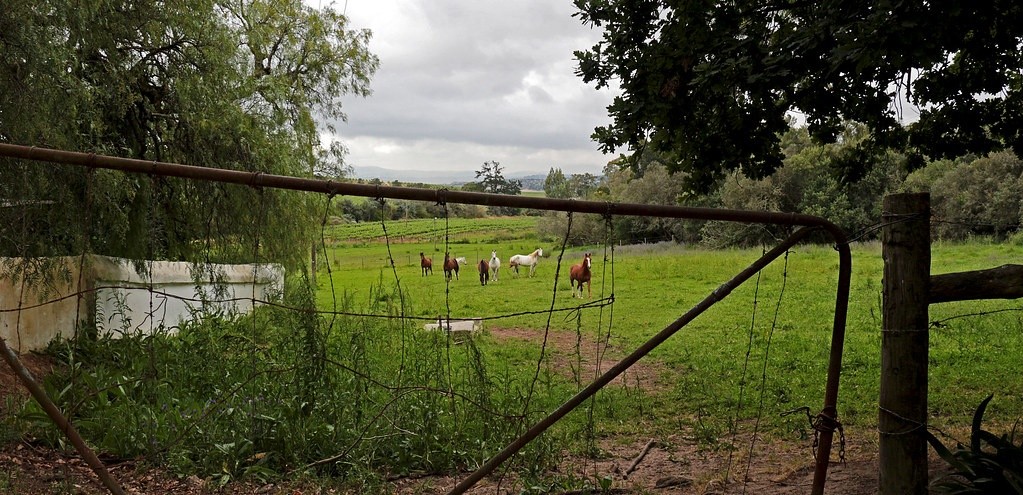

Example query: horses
[420,252,433,277]
[489,250,500,282]
[570,253,591,300]
[477,259,489,286]
[509,248,543,279]
[444,251,467,282]
